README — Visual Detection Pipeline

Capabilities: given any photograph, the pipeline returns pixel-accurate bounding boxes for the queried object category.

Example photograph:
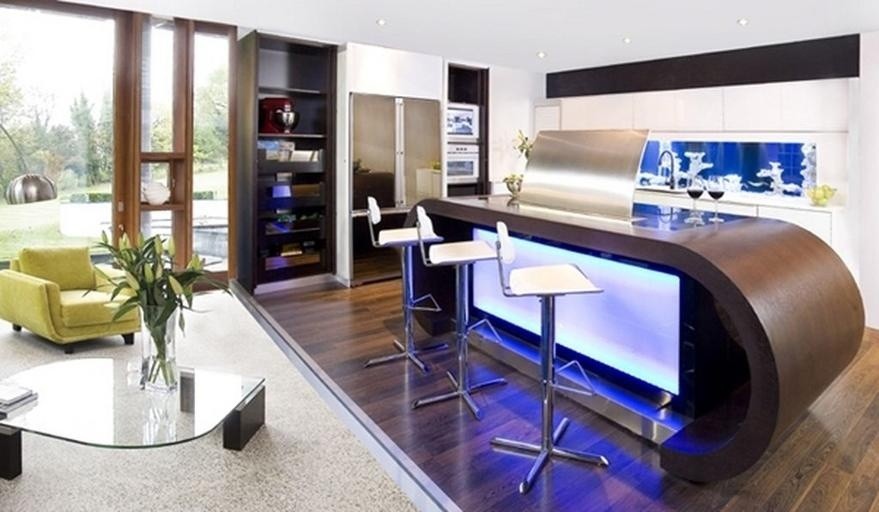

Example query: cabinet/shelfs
[238,29,332,296]
[632,187,834,247]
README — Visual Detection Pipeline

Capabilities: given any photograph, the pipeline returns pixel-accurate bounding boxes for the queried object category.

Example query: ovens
[445,101,479,186]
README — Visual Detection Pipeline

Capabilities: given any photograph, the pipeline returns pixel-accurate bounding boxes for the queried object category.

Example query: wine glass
[685,174,704,225]
[706,175,725,222]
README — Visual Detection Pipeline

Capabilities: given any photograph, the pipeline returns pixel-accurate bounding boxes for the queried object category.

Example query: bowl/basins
[801,189,836,207]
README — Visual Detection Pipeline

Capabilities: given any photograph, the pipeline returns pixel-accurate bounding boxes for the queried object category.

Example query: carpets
[1,291,418,511]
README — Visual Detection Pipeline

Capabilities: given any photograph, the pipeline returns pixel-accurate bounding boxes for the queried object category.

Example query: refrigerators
[346,92,444,287]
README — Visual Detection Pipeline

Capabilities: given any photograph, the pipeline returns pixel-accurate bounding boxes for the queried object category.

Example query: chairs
[482,219,611,496]
[364,188,455,382]
[2,245,144,356]
[408,203,509,422]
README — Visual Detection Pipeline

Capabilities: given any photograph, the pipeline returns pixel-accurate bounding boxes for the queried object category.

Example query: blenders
[261,96,300,134]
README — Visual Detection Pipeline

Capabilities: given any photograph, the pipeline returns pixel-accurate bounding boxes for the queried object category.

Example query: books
[261,139,324,162]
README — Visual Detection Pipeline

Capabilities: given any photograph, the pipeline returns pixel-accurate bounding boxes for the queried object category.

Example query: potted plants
[84,224,210,390]
